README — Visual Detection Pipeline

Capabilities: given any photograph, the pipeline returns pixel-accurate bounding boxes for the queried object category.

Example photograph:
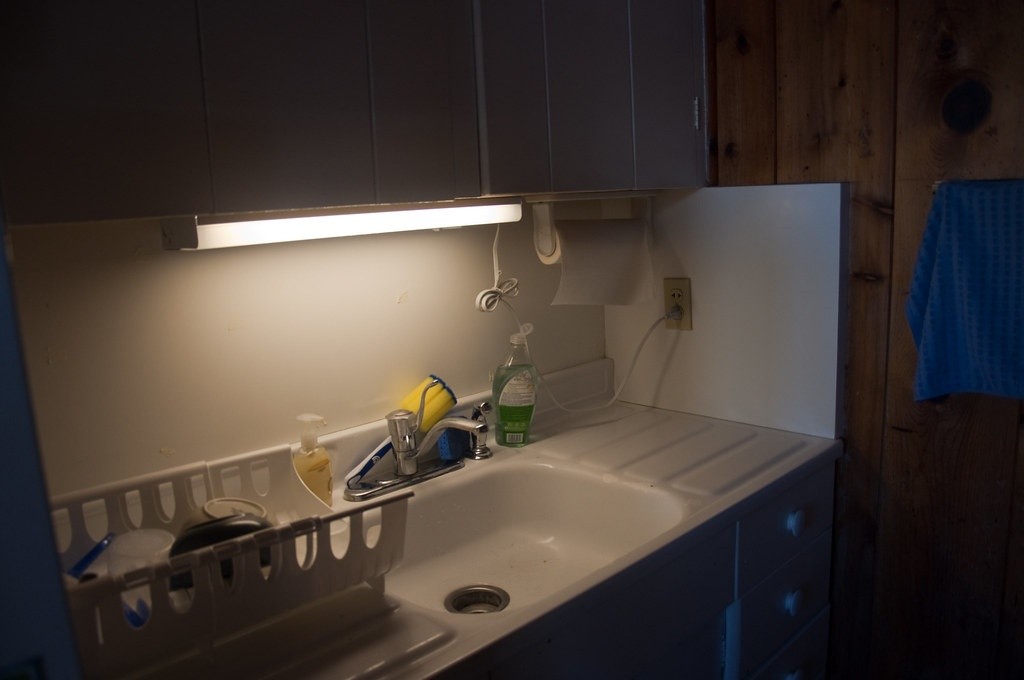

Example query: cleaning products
[492,322,538,449]
[294,414,333,508]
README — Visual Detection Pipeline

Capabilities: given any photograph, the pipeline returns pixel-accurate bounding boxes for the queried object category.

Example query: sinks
[357,452,693,622]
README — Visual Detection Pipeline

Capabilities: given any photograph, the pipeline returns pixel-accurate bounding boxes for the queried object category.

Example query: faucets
[384,378,489,477]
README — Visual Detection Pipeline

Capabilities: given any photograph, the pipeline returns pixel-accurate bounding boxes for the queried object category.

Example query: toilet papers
[536,221,654,308]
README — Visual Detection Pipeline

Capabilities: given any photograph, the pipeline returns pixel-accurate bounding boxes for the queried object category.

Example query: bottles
[108,528,176,574]
[491,321,540,447]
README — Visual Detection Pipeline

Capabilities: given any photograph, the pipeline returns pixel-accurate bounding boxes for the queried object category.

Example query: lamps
[159,196,525,253]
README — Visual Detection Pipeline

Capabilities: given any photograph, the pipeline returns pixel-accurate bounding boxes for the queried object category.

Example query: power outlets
[663,278,692,332]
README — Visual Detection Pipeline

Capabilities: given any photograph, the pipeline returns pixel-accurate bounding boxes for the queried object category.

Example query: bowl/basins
[179,496,277,536]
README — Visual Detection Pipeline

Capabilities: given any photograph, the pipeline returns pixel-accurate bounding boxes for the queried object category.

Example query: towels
[907,177,1024,402]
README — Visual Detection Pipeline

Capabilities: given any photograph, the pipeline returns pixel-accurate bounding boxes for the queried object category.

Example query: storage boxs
[45,443,416,680]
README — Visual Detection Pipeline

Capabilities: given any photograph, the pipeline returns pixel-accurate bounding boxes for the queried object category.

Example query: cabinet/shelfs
[490,438,845,680]
[0,0,710,227]
